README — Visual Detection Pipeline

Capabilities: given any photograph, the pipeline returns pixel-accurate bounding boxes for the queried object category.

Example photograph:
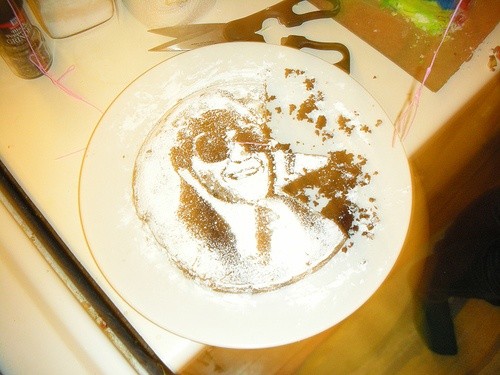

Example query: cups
[26,0,115,40]
[122,0,203,32]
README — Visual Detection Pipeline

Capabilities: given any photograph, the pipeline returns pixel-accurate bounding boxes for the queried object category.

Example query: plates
[77,41,413,352]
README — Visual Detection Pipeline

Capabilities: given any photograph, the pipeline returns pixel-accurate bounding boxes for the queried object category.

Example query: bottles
[0,0,53,81]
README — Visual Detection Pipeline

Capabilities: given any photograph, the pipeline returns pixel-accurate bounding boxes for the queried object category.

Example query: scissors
[147,0,351,79]
